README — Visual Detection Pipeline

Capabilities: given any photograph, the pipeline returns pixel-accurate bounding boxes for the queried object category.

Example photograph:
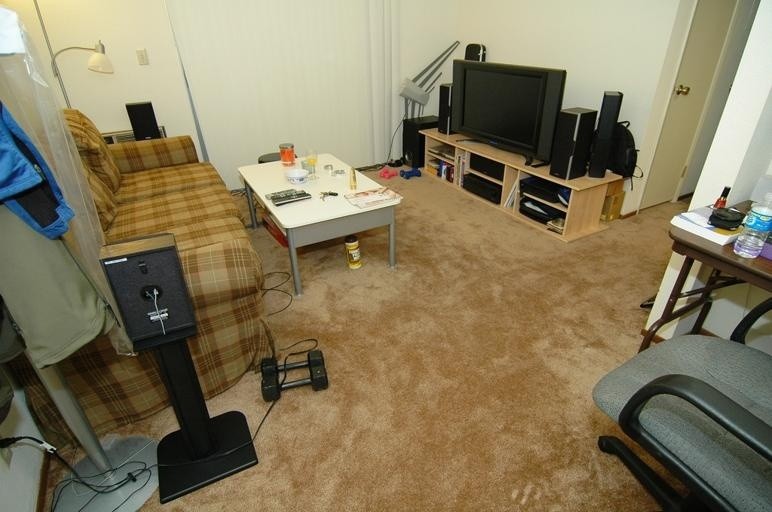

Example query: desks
[637,200,772,351]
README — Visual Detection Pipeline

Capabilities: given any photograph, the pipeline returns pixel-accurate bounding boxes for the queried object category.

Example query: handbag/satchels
[606,121,640,177]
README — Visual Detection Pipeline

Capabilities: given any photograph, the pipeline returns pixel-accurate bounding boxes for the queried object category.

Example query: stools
[258,151,297,163]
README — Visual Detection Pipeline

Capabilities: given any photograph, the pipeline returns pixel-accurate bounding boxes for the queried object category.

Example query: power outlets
[0,436,14,468]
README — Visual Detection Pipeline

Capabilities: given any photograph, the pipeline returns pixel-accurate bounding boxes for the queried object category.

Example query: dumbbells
[380,167,421,179]
[260,350,328,401]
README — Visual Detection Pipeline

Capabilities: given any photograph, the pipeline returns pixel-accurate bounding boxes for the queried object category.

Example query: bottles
[302,158,315,174]
[731,194,772,259]
[349,167,357,190]
[345,236,361,269]
[713,186,731,209]
[439,160,454,183]
[279,143,296,167]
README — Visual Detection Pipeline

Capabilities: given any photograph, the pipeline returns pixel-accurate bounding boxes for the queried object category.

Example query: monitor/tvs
[451,59,566,165]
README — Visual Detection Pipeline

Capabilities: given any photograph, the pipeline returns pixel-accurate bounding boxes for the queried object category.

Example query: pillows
[61,108,121,194]
[39,134,118,231]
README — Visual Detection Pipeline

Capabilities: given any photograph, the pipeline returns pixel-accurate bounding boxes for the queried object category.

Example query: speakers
[125,101,161,141]
[438,84,458,135]
[589,91,623,179]
[550,108,598,180]
[98,233,196,343]
[465,44,486,62]
[403,115,438,169]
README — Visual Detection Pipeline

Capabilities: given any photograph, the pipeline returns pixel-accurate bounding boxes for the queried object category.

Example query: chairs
[591,297,772,512]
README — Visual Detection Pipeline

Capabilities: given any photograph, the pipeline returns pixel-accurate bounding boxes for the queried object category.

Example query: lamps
[50,39,114,77]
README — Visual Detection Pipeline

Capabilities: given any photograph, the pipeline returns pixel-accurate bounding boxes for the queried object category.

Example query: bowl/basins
[285,168,310,184]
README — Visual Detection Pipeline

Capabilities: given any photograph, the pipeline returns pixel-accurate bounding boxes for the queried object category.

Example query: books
[670,203,744,246]
[344,186,403,209]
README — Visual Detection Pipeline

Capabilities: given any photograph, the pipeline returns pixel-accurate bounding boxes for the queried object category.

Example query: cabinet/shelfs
[419,128,623,243]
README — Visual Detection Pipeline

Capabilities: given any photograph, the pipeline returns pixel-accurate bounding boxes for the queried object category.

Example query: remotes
[272,193,312,206]
[265,189,296,199]
[272,190,306,200]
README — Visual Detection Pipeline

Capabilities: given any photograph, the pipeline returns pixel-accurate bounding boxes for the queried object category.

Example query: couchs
[14,108,263,450]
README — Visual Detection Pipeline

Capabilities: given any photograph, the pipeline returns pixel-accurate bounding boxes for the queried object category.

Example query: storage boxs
[600,179,626,222]
